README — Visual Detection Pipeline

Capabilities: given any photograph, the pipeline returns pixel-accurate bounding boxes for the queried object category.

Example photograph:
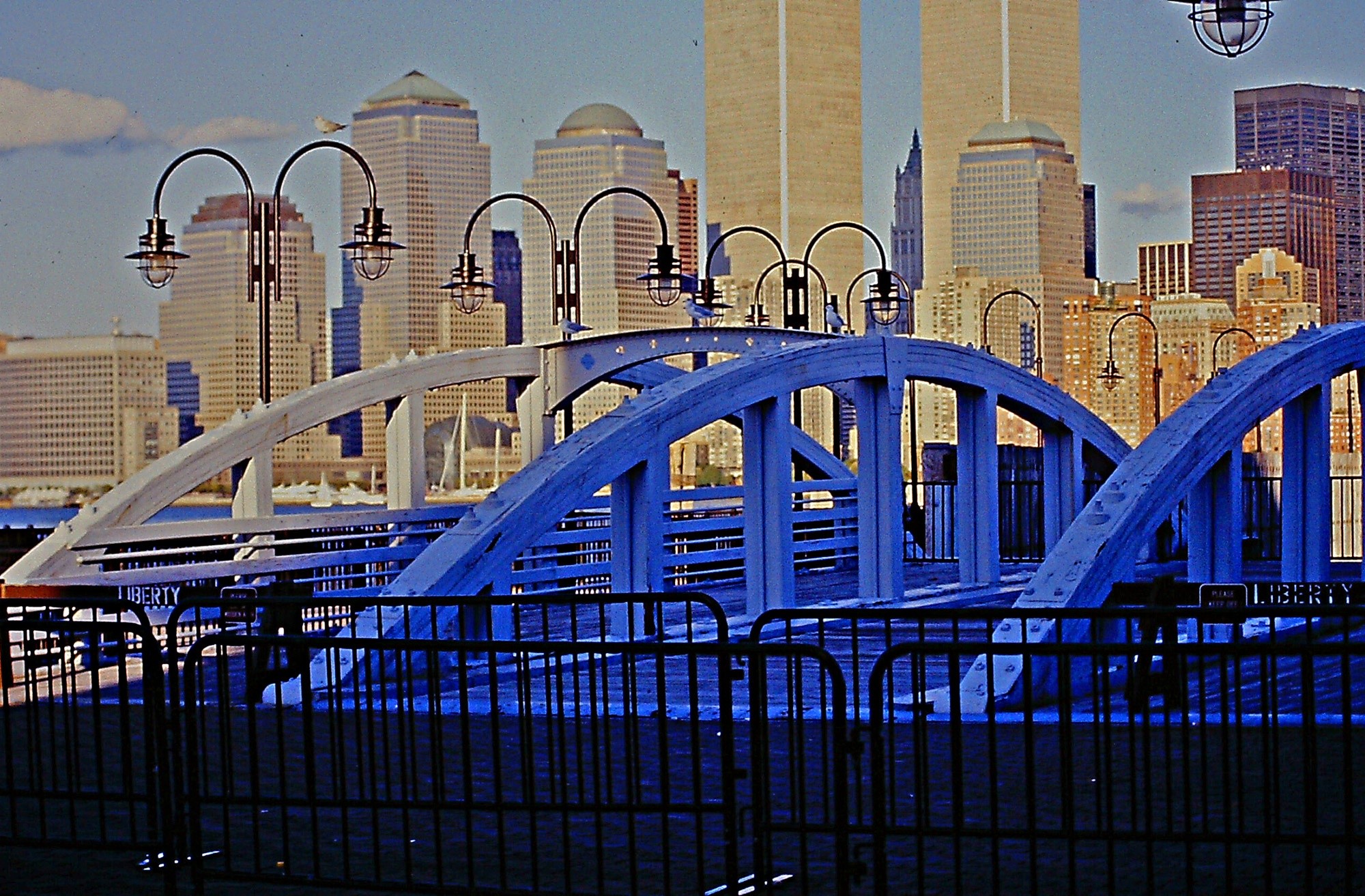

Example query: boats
[4,525,394,692]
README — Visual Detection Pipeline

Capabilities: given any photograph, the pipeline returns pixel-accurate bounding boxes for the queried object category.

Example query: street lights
[123,138,407,409]
[976,285,1042,386]
[700,218,906,510]
[1096,309,1162,430]
[442,182,688,442]
